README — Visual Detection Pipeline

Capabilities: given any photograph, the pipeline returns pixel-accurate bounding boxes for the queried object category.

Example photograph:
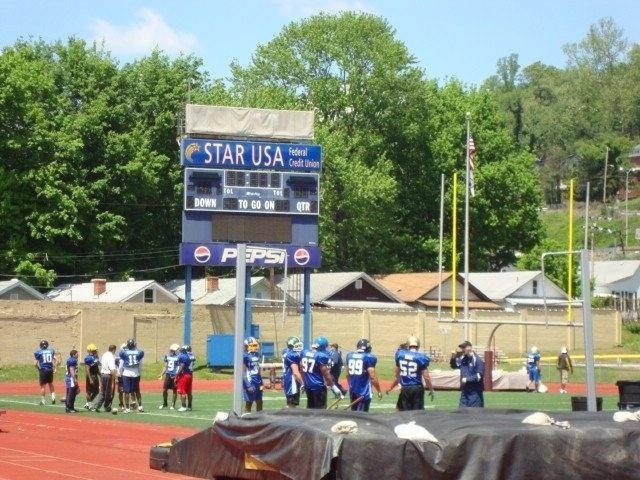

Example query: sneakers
[67,402,144,413]
[159,404,192,412]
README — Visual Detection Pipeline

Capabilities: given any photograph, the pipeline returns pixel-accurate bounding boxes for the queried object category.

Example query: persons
[344,338,384,413]
[394,335,435,410]
[525,346,542,393]
[385,343,408,396]
[33,338,196,415]
[242,336,266,414]
[278,336,346,409]
[450,341,487,407]
[554,346,575,393]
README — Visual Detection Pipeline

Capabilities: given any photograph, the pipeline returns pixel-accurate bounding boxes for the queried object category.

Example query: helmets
[127,339,136,349]
[357,339,372,352]
[406,336,420,349]
[39,340,49,349]
[170,344,192,353]
[244,337,259,353]
[87,344,98,354]
[287,336,328,351]
[560,347,567,354]
[530,346,539,354]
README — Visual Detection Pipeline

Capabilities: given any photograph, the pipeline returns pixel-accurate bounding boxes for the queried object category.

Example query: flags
[465,126,479,198]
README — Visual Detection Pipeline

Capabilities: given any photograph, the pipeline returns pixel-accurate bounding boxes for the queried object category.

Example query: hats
[330,344,339,348]
[458,341,472,348]
